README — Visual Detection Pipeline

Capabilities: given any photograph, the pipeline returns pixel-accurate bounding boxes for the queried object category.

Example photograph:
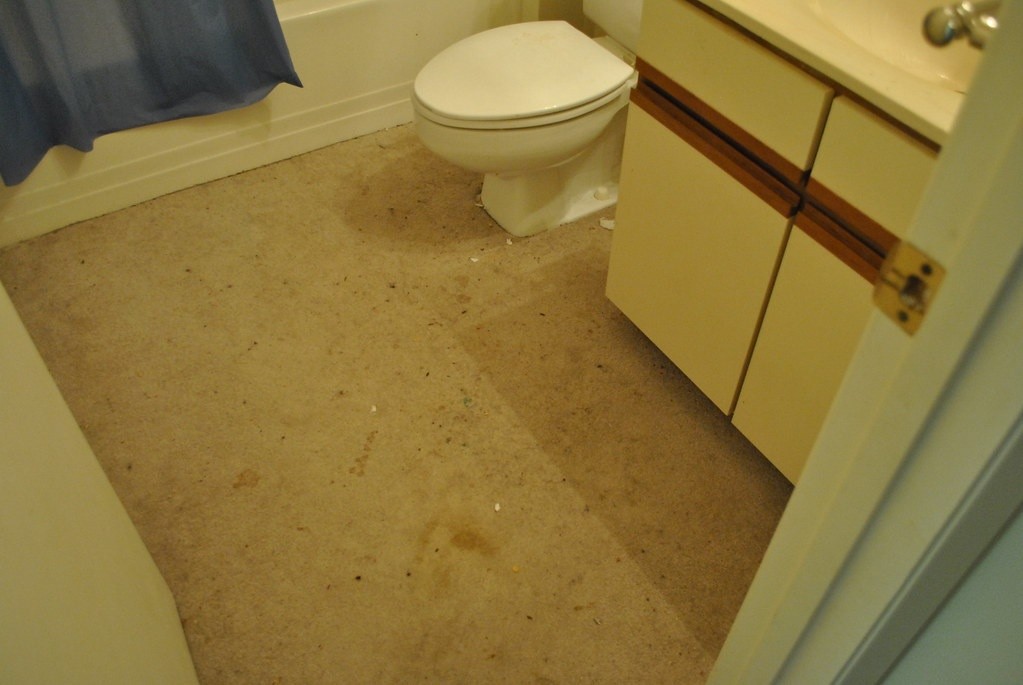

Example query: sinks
[805,0,997,95]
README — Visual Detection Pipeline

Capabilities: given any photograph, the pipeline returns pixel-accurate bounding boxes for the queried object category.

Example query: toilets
[413,18,639,239]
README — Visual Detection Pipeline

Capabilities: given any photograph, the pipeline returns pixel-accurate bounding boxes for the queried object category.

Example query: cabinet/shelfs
[581,1,941,487]
